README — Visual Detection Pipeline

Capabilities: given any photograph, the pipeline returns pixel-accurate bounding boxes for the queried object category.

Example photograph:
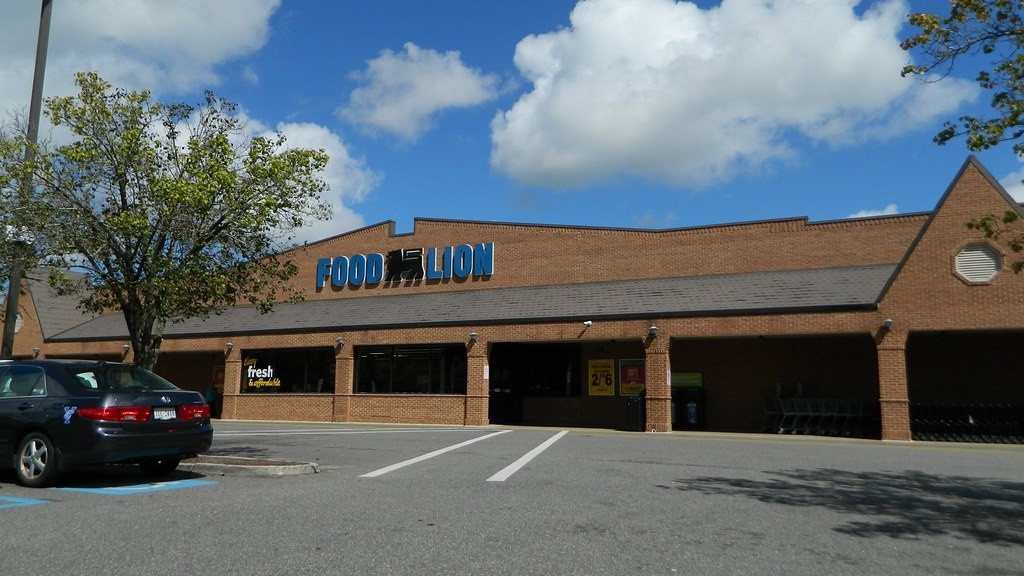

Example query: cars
[0,359,213,489]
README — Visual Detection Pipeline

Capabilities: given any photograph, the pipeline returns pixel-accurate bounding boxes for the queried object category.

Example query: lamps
[336,337,344,346]
[33,348,40,355]
[470,333,477,343]
[884,318,893,331]
[122,345,130,351]
[649,323,659,338]
[226,343,233,351]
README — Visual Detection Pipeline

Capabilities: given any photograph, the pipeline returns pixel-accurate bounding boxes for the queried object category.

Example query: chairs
[3,374,34,396]
